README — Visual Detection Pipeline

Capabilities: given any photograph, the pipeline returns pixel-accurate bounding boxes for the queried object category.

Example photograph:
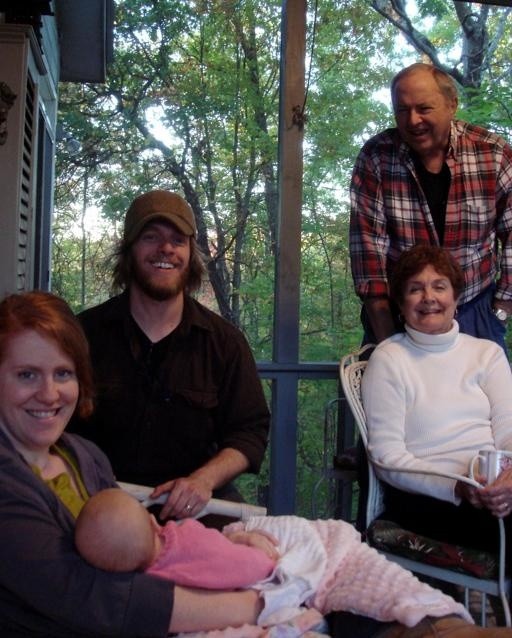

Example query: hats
[124,191,198,237]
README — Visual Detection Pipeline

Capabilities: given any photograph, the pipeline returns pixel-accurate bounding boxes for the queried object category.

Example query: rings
[503,502,509,510]
[186,505,192,510]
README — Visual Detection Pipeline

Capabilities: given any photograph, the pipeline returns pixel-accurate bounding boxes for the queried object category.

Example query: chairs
[338,342,507,628]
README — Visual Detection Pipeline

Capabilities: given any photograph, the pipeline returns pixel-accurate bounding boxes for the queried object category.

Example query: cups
[468,449,512,487]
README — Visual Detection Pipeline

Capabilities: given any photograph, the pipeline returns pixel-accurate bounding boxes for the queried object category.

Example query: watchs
[493,307,507,325]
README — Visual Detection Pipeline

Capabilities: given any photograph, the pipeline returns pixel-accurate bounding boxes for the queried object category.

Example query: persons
[75,489,275,591]
[63,191,272,523]
[348,61,512,540]
[360,241,512,627]
[0,291,511,638]
